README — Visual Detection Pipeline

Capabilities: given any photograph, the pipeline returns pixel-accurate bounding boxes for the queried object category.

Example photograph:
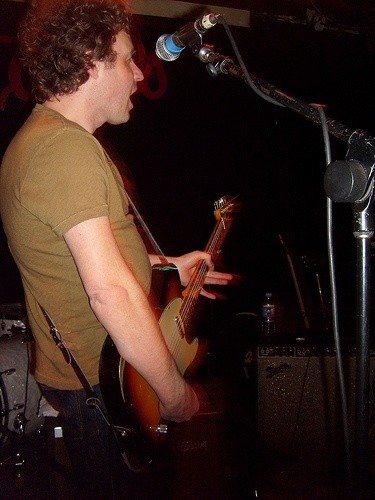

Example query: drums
[0,324,46,448]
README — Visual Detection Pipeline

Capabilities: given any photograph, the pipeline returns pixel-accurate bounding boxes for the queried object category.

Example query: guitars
[117,195,242,463]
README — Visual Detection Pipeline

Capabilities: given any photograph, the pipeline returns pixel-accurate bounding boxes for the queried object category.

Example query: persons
[0,0,243,500]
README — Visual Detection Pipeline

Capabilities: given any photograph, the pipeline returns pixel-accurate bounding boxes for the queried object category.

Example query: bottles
[261,292,276,343]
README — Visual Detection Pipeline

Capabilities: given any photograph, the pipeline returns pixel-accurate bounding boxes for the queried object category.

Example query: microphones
[155,13,221,61]
